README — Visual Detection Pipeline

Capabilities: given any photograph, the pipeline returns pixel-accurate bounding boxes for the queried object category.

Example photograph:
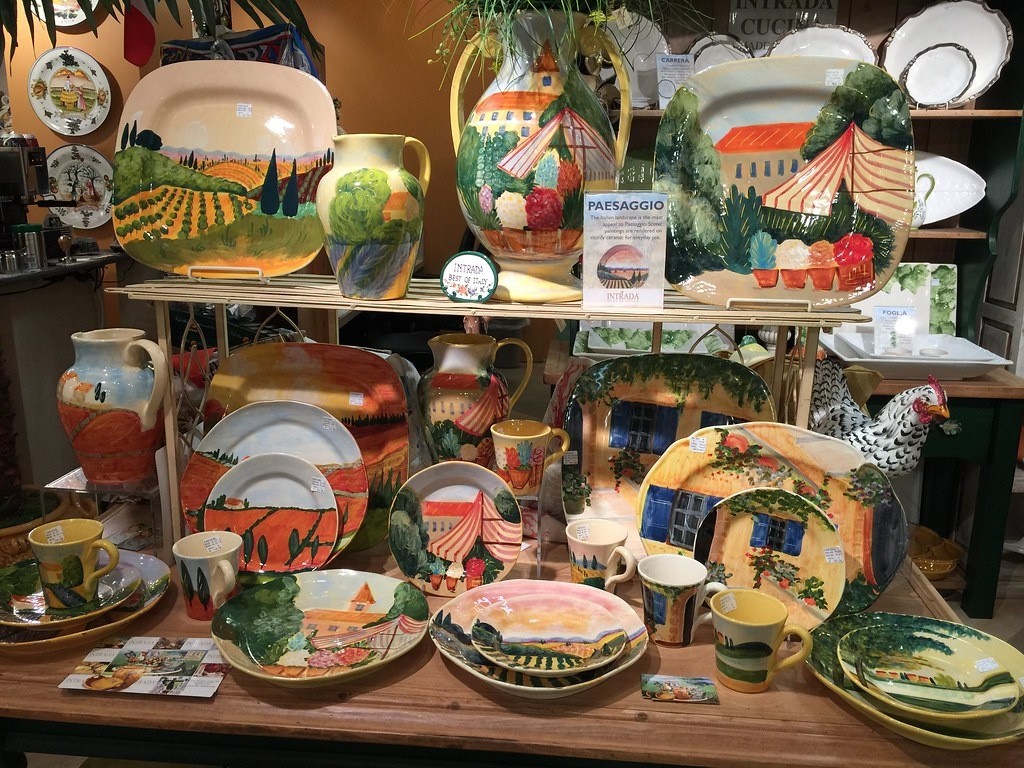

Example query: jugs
[52,326,169,488]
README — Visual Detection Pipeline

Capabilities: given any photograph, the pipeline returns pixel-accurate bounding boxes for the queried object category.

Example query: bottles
[314,132,433,302]
[416,332,534,471]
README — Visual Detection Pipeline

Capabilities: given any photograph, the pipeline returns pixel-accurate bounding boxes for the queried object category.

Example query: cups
[172,530,243,621]
[636,553,729,649]
[489,420,571,497]
[565,519,637,597]
[710,588,814,694]
[28,518,120,609]
[13,223,48,274]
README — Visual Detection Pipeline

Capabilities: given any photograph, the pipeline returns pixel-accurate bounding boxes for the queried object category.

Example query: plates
[596,0,1024,332]
[0,549,172,656]
[178,332,1024,750]
[30,0,100,27]
[40,143,114,229]
[107,60,338,277]
[26,45,111,136]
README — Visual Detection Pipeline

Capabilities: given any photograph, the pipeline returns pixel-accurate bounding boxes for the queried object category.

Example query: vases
[450,8,634,303]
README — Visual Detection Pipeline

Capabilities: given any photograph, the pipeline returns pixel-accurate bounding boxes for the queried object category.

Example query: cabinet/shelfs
[0,108,1024,768]
